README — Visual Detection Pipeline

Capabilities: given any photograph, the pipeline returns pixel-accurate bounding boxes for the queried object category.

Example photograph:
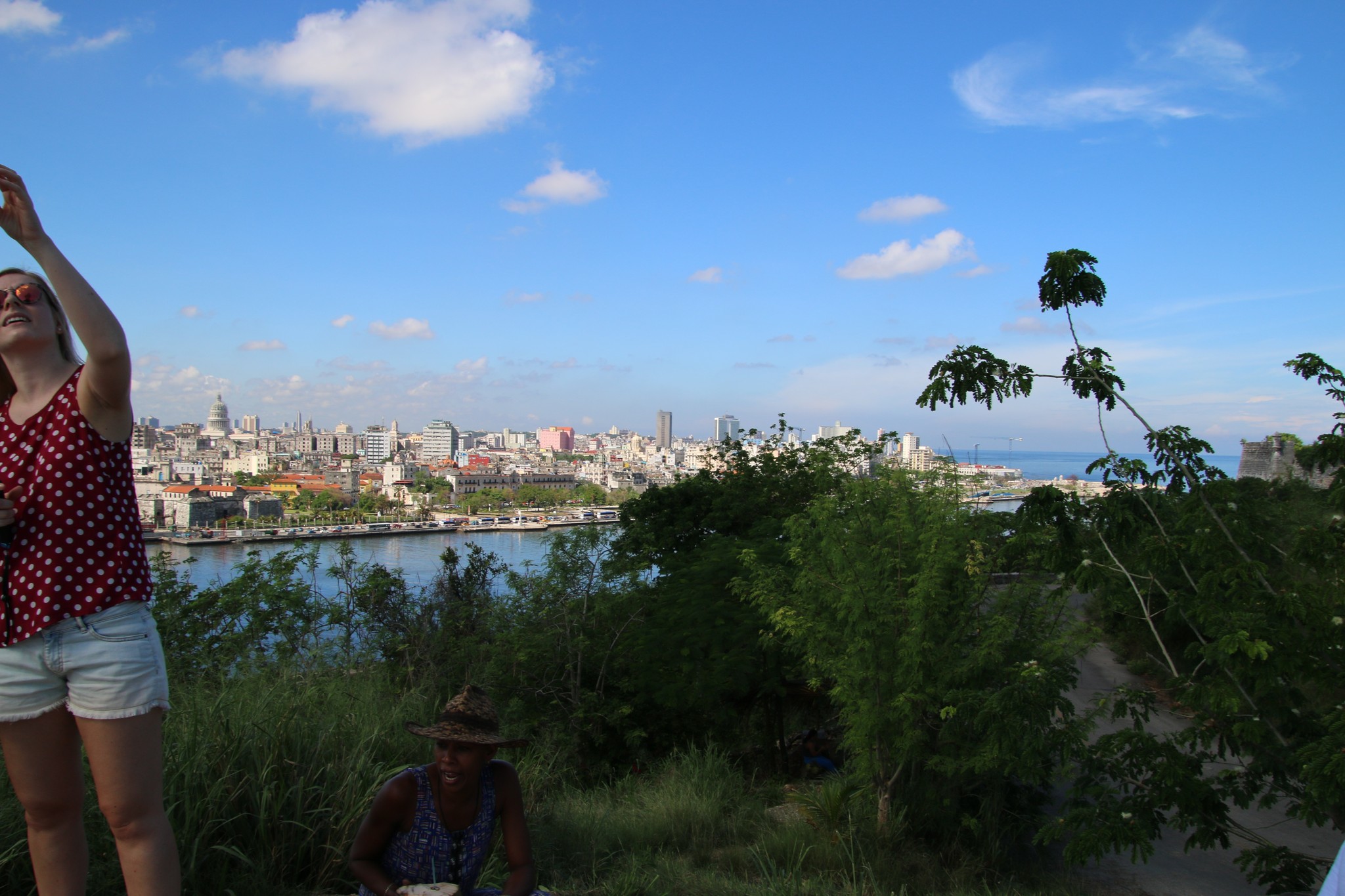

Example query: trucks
[264,528,278,535]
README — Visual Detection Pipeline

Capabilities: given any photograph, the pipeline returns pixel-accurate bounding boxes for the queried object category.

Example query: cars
[528,515,579,522]
[330,528,342,533]
[461,522,470,526]
[319,529,329,533]
[472,521,479,525]
[390,521,439,528]
[285,525,355,535]
[286,531,297,535]
[307,530,316,534]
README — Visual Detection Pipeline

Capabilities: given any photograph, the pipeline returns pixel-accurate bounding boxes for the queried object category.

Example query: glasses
[0,282,58,312]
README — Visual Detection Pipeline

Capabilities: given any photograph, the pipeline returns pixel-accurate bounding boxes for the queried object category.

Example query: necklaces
[436,770,479,829]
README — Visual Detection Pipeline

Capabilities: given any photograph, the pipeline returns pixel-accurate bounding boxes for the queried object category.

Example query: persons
[0,164,184,896]
[347,681,535,896]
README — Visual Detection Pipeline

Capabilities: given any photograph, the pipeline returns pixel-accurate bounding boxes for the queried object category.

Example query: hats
[407,684,529,745]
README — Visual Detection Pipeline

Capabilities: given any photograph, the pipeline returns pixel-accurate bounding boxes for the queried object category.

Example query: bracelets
[383,882,395,896]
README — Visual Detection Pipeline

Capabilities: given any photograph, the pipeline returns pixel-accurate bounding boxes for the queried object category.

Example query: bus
[495,517,511,524]
[512,516,528,523]
[364,522,391,531]
[446,517,469,525]
[596,511,617,519]
[440,520,456,527]
[478,517,495,525]
[580,513,595,520]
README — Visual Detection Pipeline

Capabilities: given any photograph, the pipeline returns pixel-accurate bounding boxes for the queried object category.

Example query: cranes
[993,437,1023,468]
